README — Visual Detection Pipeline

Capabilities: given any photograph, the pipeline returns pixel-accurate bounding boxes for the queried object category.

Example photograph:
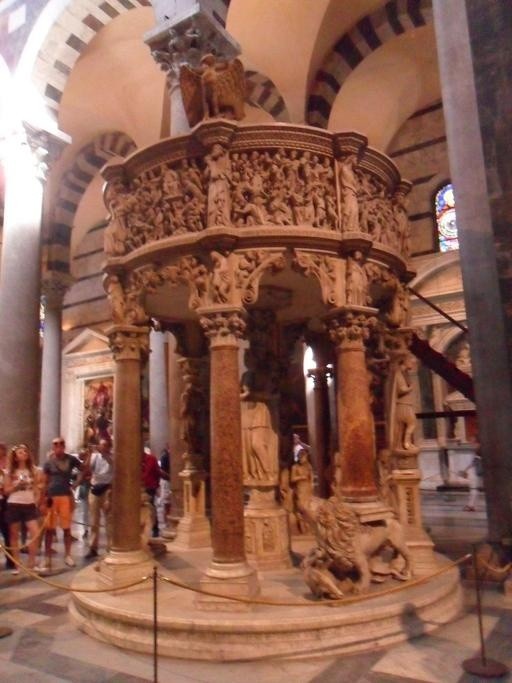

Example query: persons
[238,369,273,480]
[374,449,400,521]
[292,432,310,463]
[457,449,484,512]
[0,436,113,576]
[391,353,418,453]
[290,449,314,536]
[140,442,170,538]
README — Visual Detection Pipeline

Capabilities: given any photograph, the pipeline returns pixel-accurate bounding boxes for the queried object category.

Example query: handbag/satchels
[92,482,108,495]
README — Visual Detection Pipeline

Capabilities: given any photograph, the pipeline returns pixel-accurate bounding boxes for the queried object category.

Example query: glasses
[52,441,64,445]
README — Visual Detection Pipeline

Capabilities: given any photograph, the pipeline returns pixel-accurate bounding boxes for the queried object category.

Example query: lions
[312,499,415,594]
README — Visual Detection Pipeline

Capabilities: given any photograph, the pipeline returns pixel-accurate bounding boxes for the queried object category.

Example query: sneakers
[11,535,78,575]
[84,549,97,559]
